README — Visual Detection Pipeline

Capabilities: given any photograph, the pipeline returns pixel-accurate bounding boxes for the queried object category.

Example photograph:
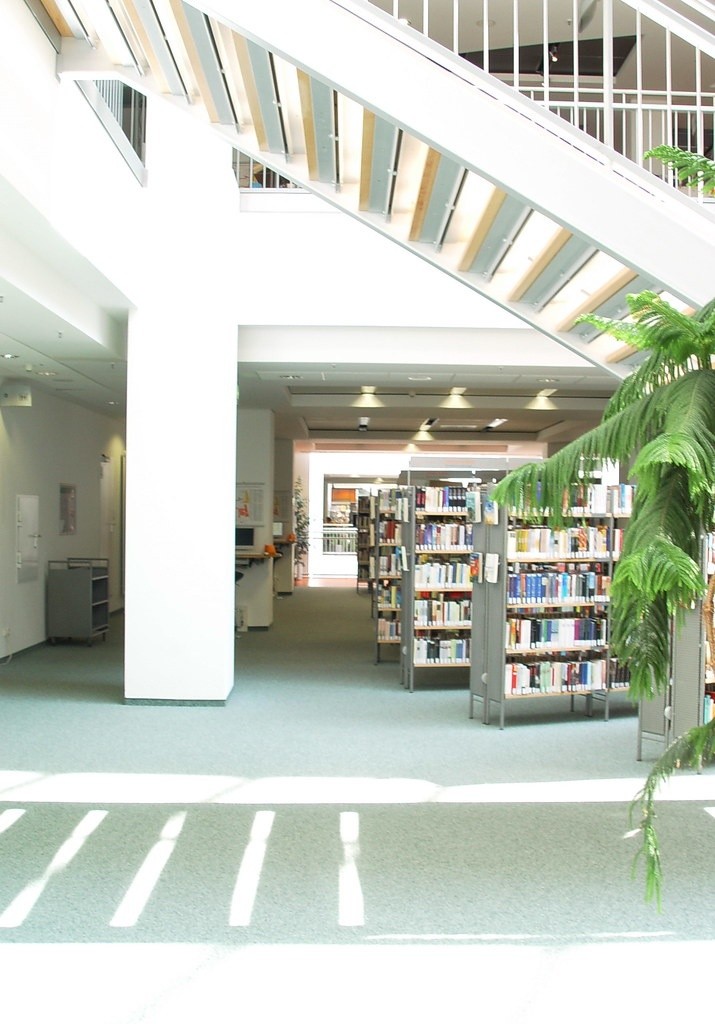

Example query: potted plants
[291,476,309,588]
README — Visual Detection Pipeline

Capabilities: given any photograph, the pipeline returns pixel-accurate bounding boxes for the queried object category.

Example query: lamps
[348,386,561,434]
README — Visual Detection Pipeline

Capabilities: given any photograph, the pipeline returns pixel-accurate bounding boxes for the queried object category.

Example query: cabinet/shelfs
[45,556,110,647]
[356,470,715,730]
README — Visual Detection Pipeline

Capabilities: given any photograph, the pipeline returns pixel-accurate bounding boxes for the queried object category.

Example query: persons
[325,517,335,549]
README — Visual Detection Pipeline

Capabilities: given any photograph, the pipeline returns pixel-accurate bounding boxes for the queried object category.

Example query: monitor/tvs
[272,521,283,538]
[235,525,254,551]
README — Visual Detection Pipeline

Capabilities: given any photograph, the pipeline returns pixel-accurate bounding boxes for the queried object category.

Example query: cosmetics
[704,530,715,727]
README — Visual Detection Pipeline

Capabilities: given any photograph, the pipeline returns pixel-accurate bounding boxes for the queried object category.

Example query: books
[505,482,637,696]
[358,490,410,643]
[413,486,499,665]
